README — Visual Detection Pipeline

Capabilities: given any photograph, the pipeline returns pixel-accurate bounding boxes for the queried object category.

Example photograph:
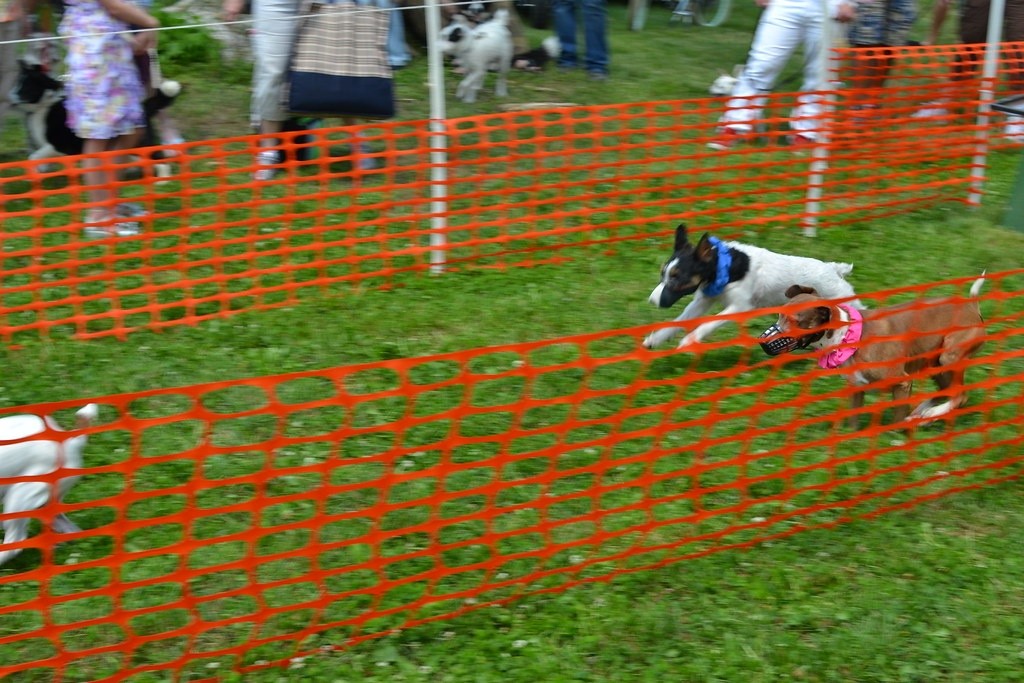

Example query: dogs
[640,220,993,443]
[438,8,514,104]
[0,54,186,188]
[0,402,102,568]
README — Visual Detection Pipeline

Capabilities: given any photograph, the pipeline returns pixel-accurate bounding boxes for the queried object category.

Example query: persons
[442,0,528,74]
[911,0,1024,141]
[705,0,858,152]
[224,1,379,184]
[849,1,911,110]
[553,1,609,79]
[58,1,161,236]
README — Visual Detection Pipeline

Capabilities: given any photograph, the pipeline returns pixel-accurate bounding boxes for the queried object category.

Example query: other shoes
[1004,112,1024,141]
[84,204,148,236]
[254,151,279,179]
[588,72,605,81]
[554,65,571,74]
[910,102,951,125]
[708,128,741,151]
[349,142,375,172]
[791,135,814,157]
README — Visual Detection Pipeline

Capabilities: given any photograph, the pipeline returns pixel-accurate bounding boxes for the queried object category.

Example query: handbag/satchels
[287,3,399,121]
